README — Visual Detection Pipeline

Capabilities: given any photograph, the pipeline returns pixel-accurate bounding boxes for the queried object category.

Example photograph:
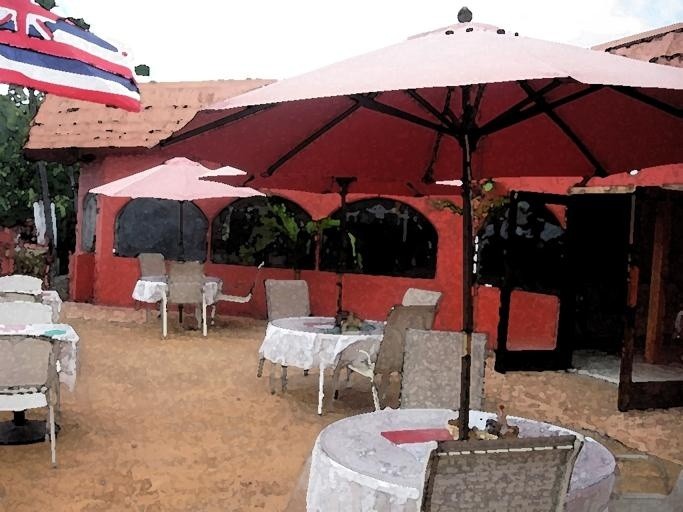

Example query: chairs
[1,275,62,467]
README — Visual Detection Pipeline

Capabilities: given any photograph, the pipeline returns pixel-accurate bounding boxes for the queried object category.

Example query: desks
[0,322,80,445]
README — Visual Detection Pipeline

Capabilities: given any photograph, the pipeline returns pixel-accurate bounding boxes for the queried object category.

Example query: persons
[15,217,38,244]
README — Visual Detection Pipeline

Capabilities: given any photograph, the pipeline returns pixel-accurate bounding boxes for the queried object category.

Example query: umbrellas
[150,3,683,442]
[0,0,142,112]
[480,163,682,280]
[88,158,268,322]
[198,164,463,401]
